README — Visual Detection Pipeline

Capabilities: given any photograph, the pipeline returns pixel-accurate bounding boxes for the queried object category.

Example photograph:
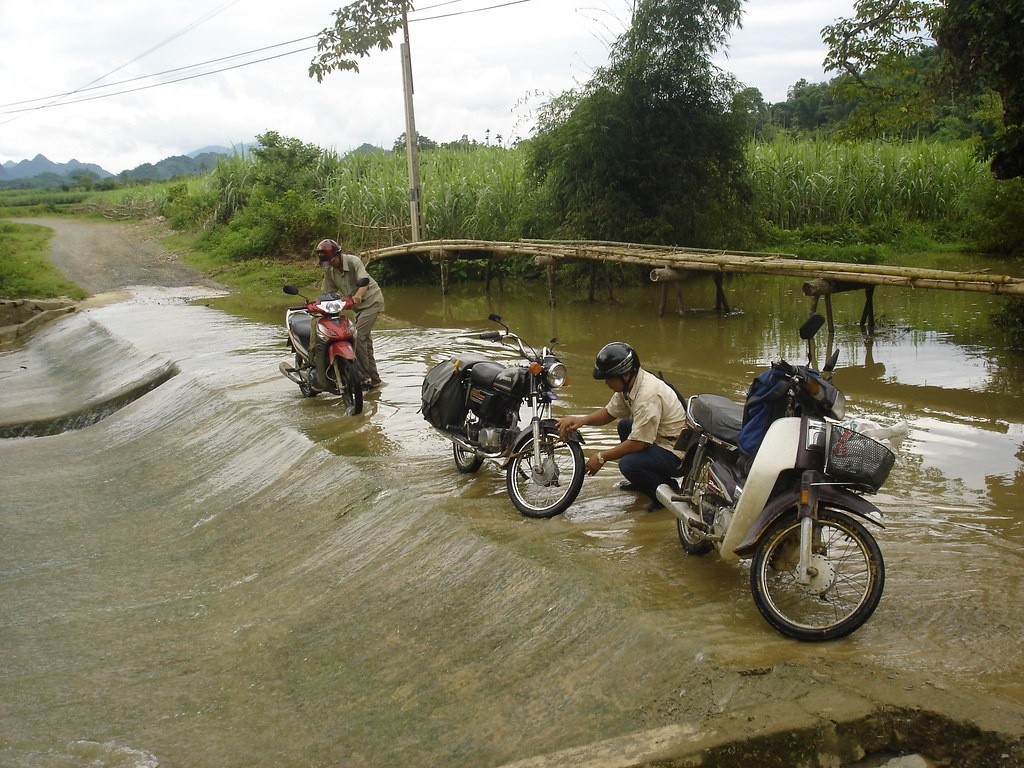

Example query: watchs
[596,451,605,465]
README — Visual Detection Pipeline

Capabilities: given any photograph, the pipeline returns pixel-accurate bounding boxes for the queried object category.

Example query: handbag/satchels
[421,359,466,429]
[735,365,820,479]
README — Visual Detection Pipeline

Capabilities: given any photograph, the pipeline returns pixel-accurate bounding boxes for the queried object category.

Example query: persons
[314,239,384,388]
[555,342,693,512]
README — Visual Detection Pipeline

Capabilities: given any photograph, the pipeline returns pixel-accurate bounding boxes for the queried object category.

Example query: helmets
[316,239,341,267]
[592,342,640,380]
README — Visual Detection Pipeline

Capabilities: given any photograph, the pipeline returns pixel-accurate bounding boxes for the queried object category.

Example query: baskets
[826,424,896,493]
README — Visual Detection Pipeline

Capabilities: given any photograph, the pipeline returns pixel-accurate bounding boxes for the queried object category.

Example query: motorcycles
[433,312,587,518]
[279,277,371,418]
[655,312,901,642]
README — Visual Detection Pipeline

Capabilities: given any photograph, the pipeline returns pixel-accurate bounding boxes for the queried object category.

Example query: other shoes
[361,378,371,385]
[372,379,382,388]
[648,501,664,511]
[620,481,635,490]
